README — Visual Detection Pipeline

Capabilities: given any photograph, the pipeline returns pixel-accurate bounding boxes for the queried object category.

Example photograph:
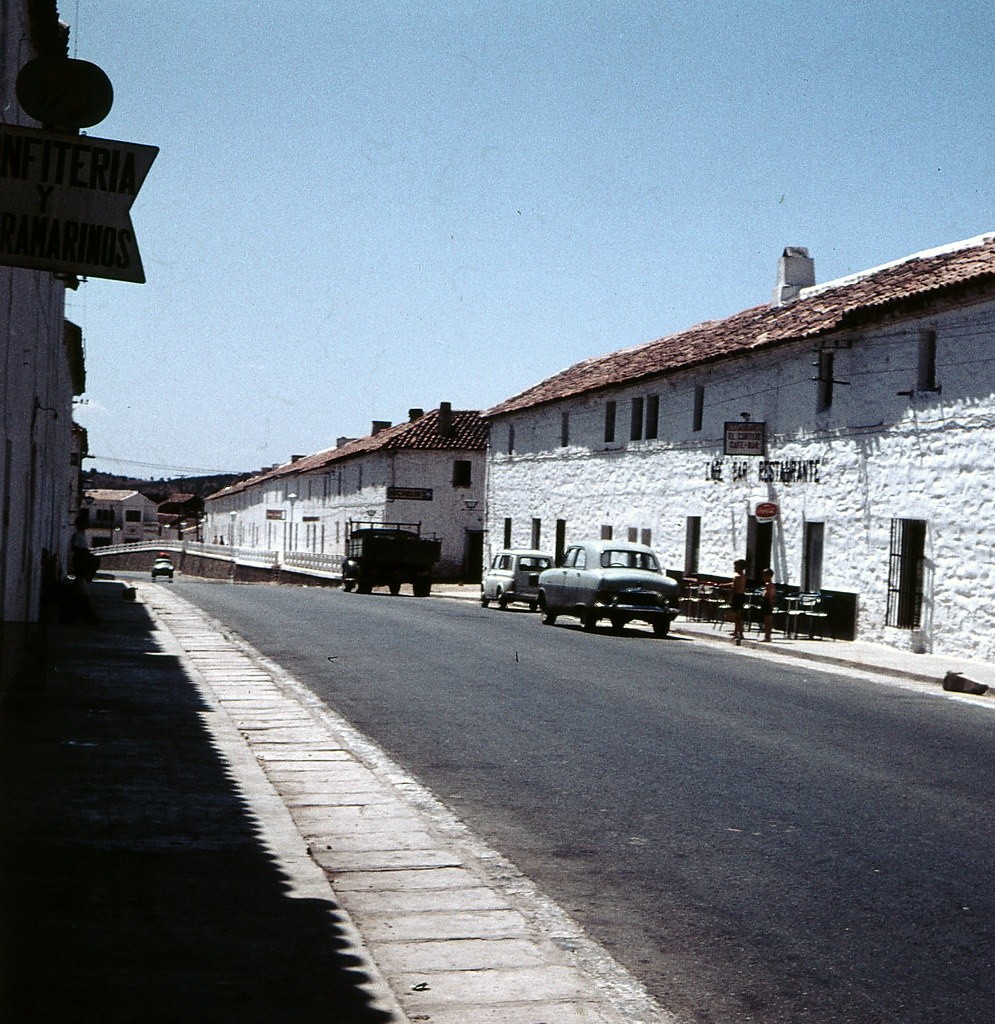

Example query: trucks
[341,518,443,596]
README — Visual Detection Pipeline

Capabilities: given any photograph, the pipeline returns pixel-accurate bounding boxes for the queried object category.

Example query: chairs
[678,576,829,641]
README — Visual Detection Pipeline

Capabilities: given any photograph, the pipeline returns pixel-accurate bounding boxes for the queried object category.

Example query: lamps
[460,498,484,523]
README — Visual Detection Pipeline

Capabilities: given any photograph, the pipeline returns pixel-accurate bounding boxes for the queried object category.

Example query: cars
[151,557,174,578]
[538,538,681,632]
[481,552,555,609]
